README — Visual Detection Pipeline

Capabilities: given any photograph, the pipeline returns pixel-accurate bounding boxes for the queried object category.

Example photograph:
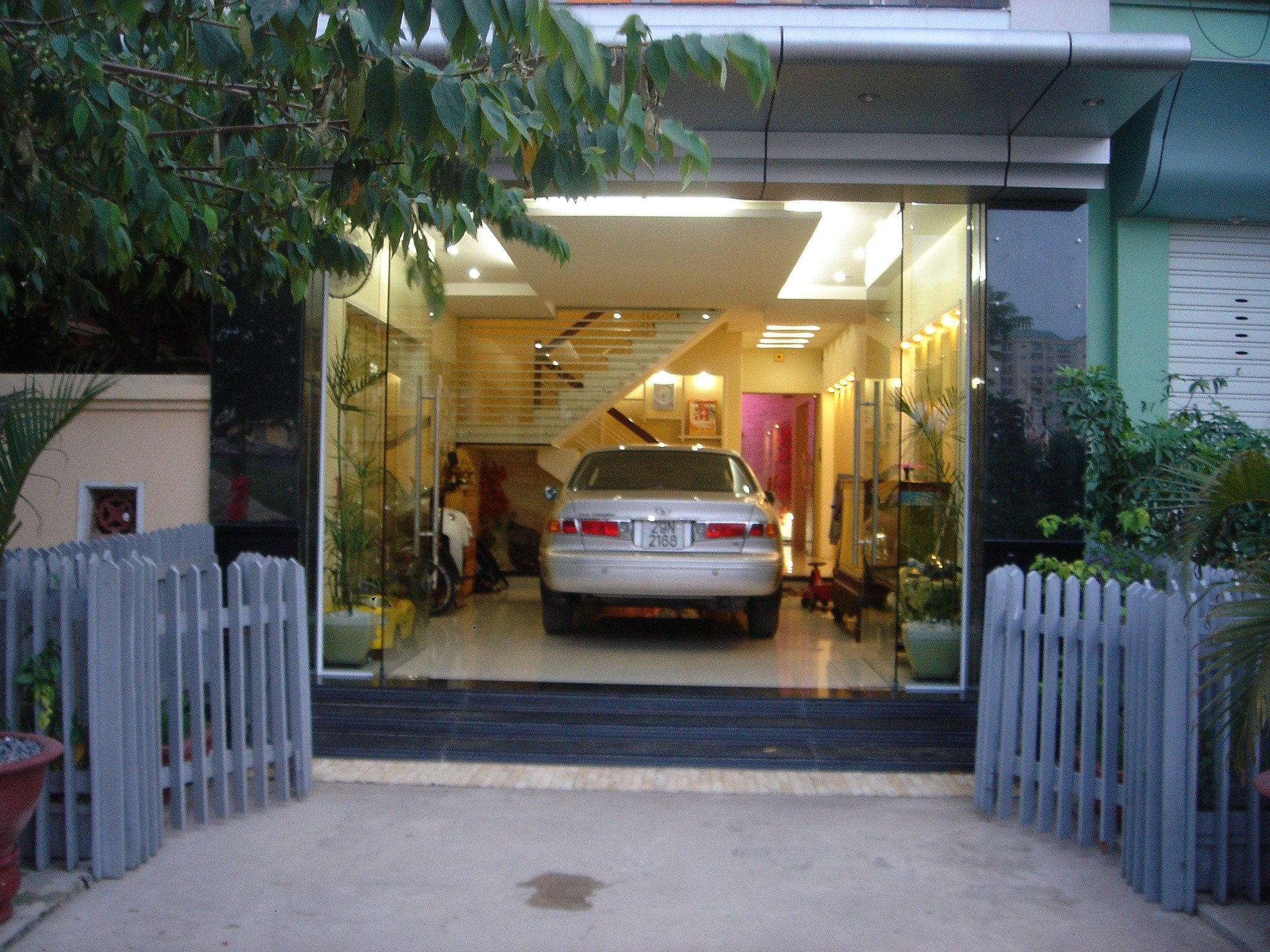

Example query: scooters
[405,448,473,616]
[886,553,962,626]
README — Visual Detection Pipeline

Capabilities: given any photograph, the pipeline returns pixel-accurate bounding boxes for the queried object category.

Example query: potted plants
[1074,731,1123,828]
[0,349,126,858]
[159,695,214,799]
[322,317,388,663]
[888,385,963,680]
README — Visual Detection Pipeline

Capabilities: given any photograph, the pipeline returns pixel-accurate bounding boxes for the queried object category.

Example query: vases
[0,842,23,922]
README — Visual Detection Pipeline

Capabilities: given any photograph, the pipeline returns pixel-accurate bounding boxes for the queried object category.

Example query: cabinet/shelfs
[838,479,961,592]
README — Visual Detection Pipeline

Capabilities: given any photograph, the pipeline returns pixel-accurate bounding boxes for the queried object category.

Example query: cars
[537,441,786,638]
